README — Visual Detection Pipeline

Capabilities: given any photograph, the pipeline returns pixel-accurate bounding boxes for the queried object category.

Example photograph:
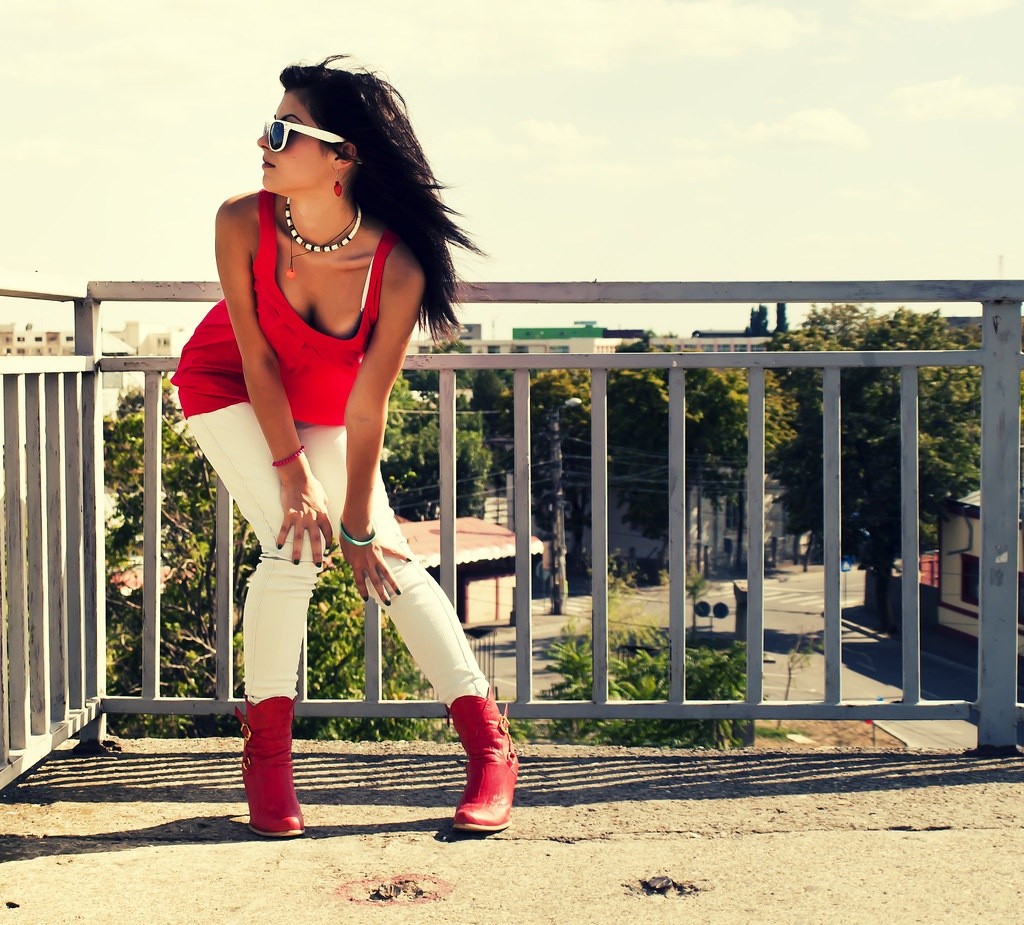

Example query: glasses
[264,118,363,164]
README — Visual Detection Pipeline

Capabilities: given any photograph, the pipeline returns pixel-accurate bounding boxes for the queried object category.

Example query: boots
[235,693,307,837]
[444,688,520,831]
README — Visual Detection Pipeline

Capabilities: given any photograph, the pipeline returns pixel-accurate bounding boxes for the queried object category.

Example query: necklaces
[285,197,361,253]
[286,212,356,279]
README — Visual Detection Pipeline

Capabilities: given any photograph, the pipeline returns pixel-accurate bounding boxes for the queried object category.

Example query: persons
[170,66,518,836]
[625,547,638,592]
[770,537,779,568]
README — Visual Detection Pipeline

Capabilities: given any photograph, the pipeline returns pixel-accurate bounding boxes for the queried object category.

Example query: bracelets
[339,515,376,546]
[273,446,305,466]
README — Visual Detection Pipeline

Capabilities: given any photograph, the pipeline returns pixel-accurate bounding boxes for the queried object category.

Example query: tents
[66,327,138,354]
